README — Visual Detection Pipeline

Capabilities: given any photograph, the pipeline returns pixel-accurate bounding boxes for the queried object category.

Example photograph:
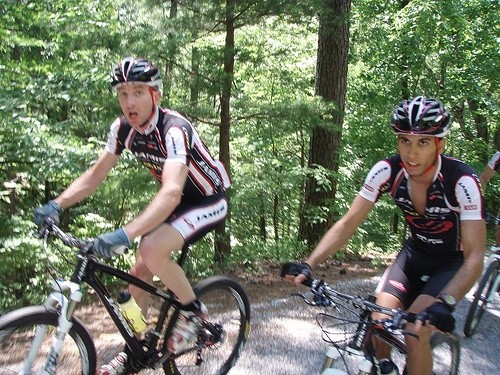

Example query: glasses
[394,115,444,132]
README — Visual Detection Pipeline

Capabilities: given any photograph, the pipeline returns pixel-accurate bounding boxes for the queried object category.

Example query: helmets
[110,58,163,96]
[391,96,450,137]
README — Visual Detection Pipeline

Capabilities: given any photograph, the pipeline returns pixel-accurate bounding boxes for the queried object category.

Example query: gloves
[92,228,133,259]
[34,200,64,235]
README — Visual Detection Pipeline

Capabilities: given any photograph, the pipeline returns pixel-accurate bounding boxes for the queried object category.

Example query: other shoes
[380,363,400,375]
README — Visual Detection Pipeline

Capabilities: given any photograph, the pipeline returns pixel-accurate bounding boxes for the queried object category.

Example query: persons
[281,94,486,375]
[479,125,500,296]
[33,58,234,375]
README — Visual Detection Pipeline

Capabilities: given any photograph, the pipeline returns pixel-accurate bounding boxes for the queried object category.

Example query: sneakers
[167,302,208,353]
[96,351,152,375]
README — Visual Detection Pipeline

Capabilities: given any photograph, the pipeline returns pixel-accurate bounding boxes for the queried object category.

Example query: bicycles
[0,218,252,375]
[463,209,500,337]
[290,274,461,374]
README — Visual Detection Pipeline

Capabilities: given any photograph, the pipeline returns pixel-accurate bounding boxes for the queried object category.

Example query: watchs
[436,292,456,308]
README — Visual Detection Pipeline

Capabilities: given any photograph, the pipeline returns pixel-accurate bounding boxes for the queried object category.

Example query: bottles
[117,291,148,334]
[379,358,399,375]
